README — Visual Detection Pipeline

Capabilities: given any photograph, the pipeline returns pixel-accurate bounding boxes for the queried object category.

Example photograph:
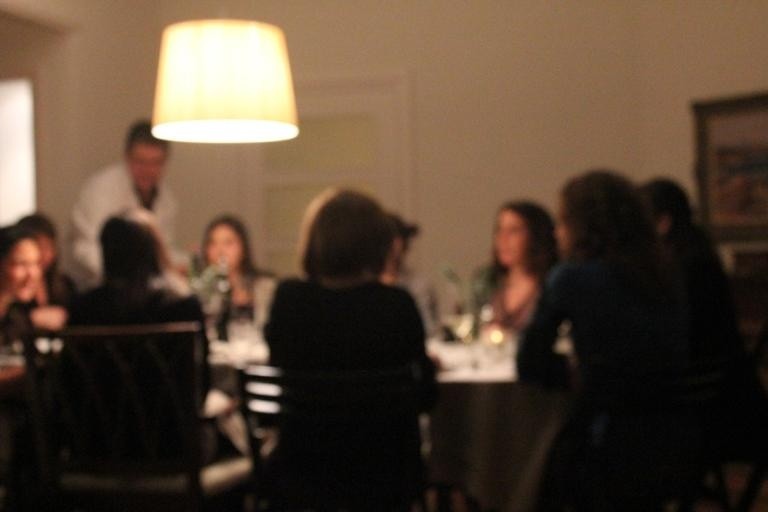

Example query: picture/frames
[690,90,768,242]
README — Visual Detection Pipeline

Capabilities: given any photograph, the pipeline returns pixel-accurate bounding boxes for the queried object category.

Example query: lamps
[150,18,301,144]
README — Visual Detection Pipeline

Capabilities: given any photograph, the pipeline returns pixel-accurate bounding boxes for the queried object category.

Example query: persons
[265,190,443,512]
[0,120,276,511]
[465,167,749,512]
